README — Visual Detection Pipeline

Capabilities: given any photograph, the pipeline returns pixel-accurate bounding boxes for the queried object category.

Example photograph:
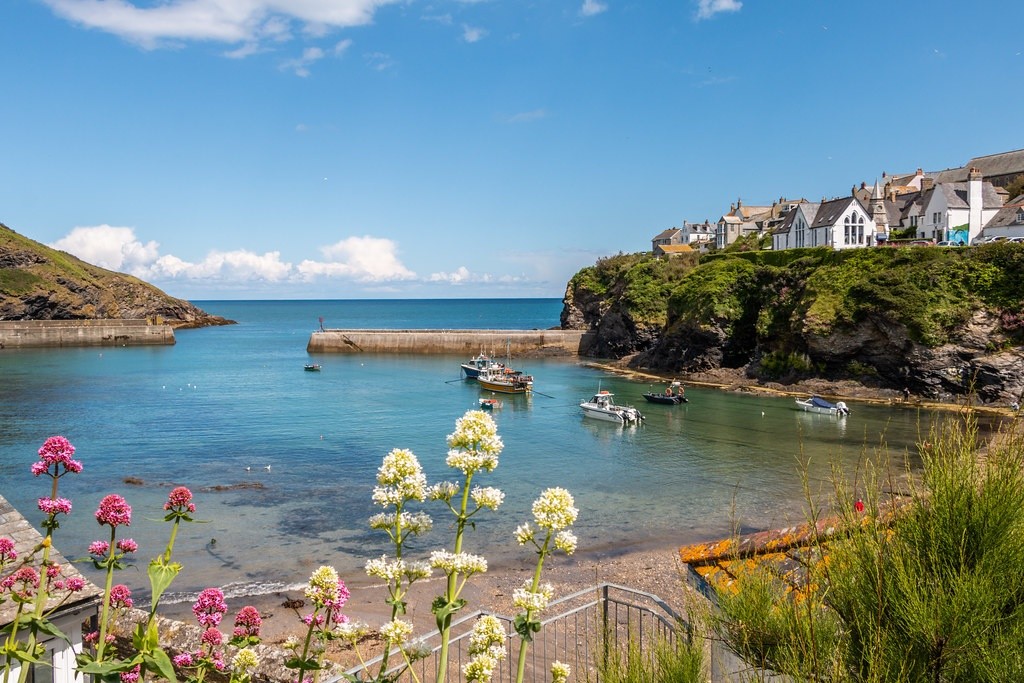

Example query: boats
[460,335,523,380]
[579,377,645,424]
[795,393,854,416]
[304,363,322,371]
[476,338,534,393]
[642,378,688,405]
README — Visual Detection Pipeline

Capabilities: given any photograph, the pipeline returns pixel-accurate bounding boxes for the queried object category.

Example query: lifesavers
[678,387,684,394]
[666,388,673,395]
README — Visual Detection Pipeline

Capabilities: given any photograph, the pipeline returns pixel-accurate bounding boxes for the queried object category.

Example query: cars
[910,240,935,246]
[974,235,1024,245]
[936,240,960,247]
